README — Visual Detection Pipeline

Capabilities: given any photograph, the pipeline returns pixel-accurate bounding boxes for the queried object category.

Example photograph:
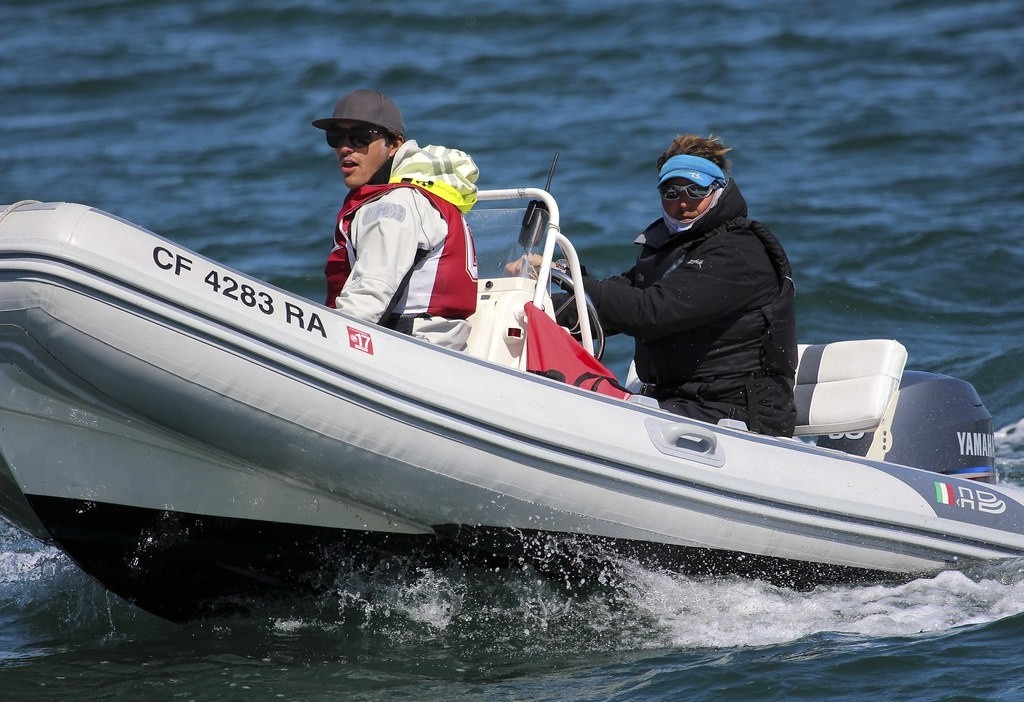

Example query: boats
[0,188,1023,622]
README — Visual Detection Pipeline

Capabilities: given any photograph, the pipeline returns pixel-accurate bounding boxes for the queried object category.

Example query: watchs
[552,259,567,286]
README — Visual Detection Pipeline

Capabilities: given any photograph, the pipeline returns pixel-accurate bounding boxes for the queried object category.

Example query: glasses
[324,126,387,148]
[658,183,720,201]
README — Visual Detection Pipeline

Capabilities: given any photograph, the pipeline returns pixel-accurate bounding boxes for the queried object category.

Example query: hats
[656,154,726,187]
[311,89,404,138]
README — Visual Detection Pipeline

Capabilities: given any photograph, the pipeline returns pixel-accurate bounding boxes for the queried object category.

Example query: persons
[505,137,799,438]
[311,90,480,351]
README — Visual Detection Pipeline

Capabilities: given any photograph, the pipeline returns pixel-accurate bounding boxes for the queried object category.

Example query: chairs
[457,190,559,373]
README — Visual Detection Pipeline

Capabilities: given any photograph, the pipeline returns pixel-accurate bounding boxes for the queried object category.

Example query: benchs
[625,337,909,464]
